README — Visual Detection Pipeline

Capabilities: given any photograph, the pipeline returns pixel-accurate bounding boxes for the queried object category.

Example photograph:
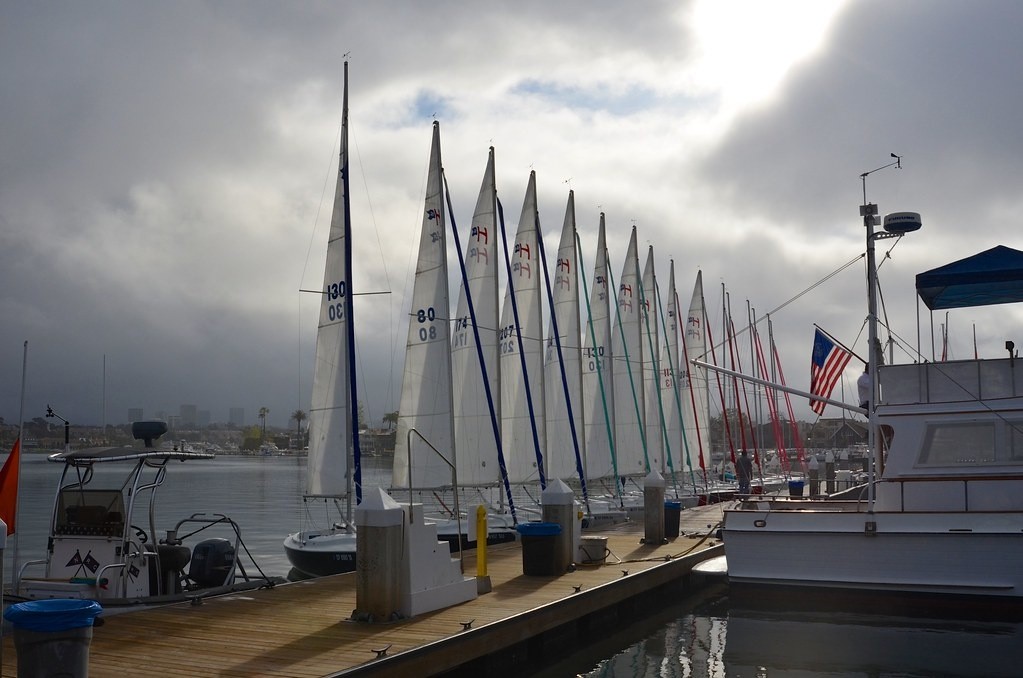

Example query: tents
[915,245,1023,363]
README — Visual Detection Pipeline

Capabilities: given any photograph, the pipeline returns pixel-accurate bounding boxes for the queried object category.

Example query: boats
[0,405,288,633]
[689,153,1023,626]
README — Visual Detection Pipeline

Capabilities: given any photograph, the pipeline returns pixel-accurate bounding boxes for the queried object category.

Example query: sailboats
[283,49,812,580]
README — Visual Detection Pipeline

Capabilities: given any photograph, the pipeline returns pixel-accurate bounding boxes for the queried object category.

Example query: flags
[809,328,853,417]
[0,438,20,536]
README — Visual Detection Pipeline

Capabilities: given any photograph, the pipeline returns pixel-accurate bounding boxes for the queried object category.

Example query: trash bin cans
[518,521,560,576]
[10,598,97,678]
[788,481,805,500]
[664,501,682,537]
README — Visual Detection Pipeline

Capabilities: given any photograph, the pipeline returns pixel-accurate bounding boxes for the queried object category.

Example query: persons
[856,361,892,452]
[736,451,754,501]
